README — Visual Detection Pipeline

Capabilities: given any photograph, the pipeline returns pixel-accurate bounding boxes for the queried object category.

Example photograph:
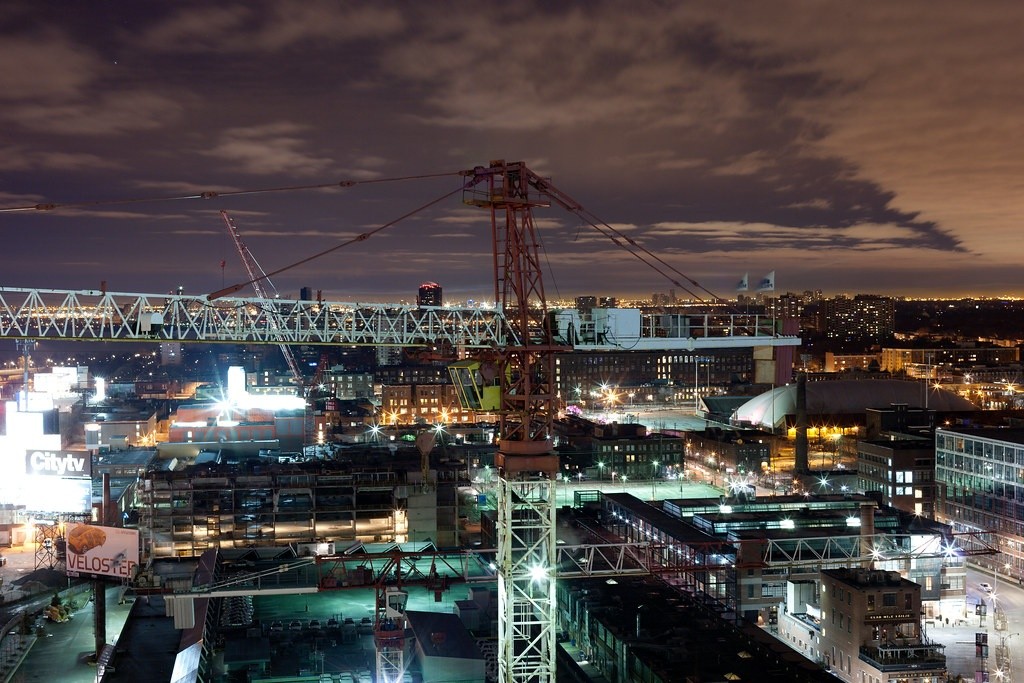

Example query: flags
[756,271,774,293]
[736,273,747,292]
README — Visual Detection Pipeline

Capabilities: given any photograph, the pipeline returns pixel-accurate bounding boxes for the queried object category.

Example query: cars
[977,583,992,592]
[42,538,52,548]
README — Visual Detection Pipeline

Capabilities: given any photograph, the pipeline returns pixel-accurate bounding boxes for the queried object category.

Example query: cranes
[0,157,807,683]
